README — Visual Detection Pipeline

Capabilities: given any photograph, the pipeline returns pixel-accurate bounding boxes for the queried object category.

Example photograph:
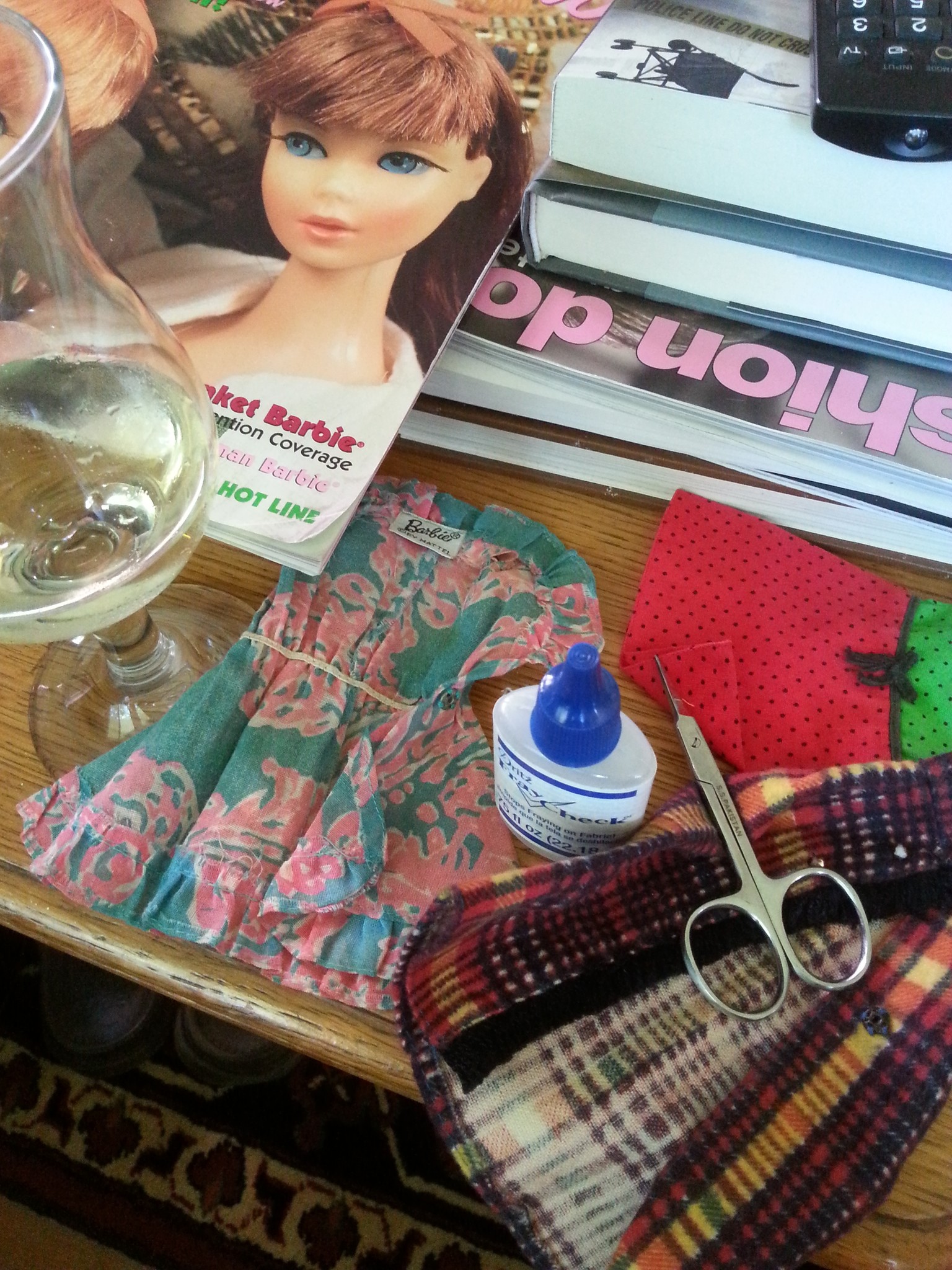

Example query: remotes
[807,1,951,162]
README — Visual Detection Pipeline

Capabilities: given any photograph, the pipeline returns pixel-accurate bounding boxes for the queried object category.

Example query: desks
[0,427,952,1270]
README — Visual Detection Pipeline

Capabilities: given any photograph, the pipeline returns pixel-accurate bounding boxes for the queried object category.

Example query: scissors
[652,653,873,1019]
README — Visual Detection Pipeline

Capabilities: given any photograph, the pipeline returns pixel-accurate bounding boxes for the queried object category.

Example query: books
[0,0,949,579]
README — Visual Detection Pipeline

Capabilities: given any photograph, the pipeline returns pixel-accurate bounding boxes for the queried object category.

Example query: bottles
[492,643,659,862]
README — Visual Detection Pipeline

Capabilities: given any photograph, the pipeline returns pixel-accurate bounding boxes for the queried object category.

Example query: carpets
[0,926,540,1270]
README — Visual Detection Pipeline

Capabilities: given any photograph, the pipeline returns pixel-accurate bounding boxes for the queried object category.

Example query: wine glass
[0,4,255,776]
